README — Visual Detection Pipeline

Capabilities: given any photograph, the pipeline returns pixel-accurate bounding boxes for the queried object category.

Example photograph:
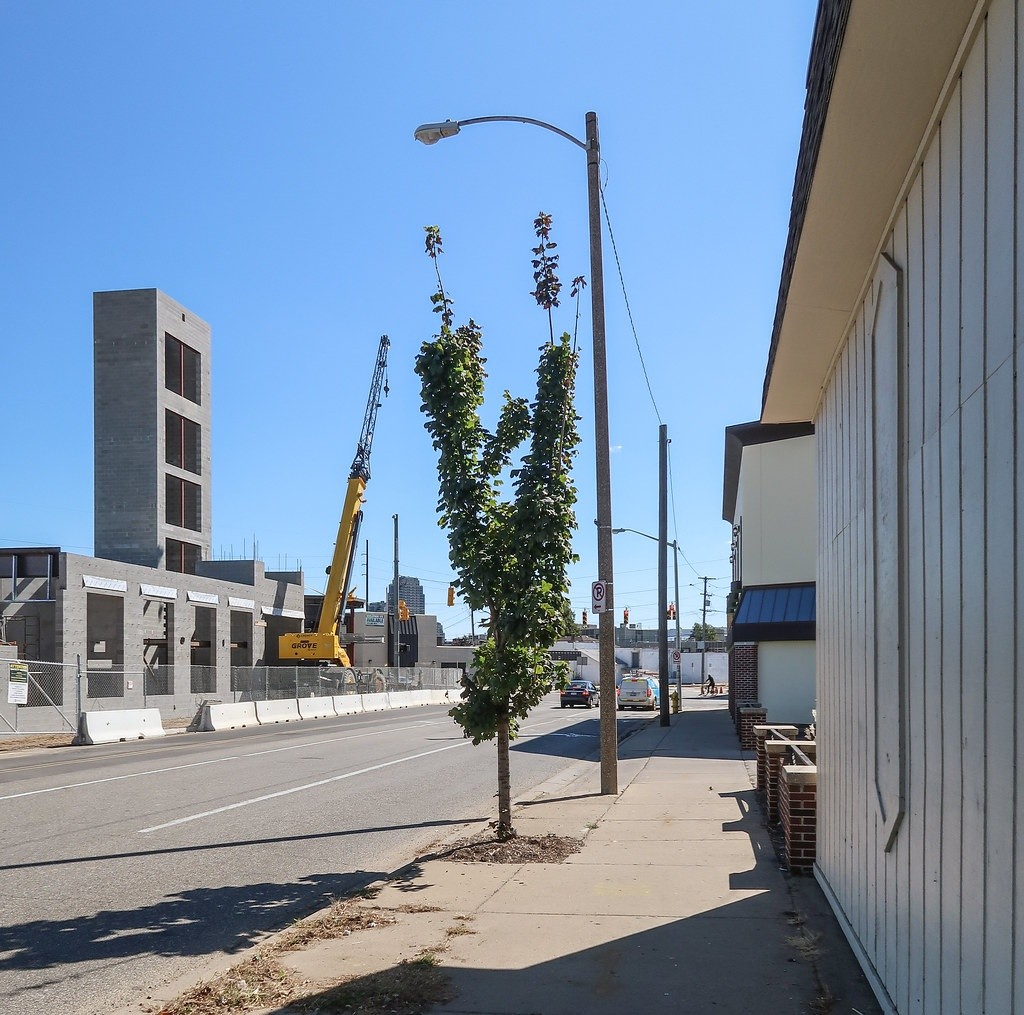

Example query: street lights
[611,528,683,711]
[414,112,618,796]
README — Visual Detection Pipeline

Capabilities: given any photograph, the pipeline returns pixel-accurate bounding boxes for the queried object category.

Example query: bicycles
[701,683,718,696]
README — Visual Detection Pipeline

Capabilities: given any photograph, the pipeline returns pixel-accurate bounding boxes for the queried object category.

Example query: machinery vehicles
[278,335,391,698]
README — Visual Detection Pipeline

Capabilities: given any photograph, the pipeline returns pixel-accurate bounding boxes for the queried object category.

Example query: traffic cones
[720,687,722,693]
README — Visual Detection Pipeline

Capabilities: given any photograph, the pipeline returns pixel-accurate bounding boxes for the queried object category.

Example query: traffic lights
[673,612,677,620]
[670,605,674,610]
[667,610,671,620]
[583,612,587,624]
[624,610,629,624]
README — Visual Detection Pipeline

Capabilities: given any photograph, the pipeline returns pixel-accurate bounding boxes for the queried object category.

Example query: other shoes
[707,691,713,694]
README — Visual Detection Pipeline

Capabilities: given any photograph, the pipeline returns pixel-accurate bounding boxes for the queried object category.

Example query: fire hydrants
[671,691,679,714]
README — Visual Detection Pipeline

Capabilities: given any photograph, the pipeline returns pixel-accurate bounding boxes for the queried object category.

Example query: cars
[616,670,660,711]
[560,681,600,709]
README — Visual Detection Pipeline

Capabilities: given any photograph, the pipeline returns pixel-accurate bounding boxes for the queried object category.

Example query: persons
[705,674,715,694]
